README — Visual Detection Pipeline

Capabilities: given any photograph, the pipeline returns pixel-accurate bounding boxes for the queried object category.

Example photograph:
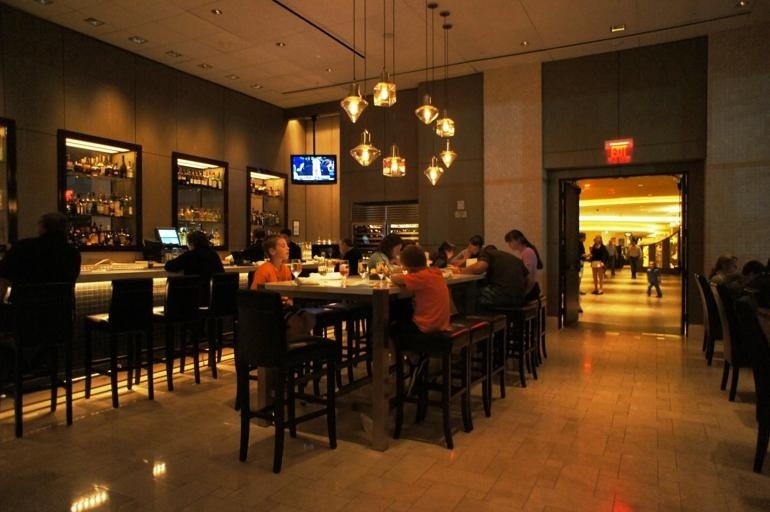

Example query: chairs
[2,288,75,438]
[195,271,239,379]
[90,279,157,407]
[154,275,202,390]
[689,271,770,472]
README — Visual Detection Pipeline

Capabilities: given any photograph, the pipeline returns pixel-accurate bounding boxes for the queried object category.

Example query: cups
[148,260,153,268]
[324,259,335,276]
[305,247,335,263]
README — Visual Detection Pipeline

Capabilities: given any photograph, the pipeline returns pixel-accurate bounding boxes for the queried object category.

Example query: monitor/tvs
[291,155,337,184]
[156,227,180,246]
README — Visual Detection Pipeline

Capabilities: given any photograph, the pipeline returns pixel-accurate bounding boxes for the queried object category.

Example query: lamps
[344,0,462,186]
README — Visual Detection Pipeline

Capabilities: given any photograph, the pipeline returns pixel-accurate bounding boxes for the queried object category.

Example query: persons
[1,213,81,388]
[163,230,226,338]
[579,232,663,298]
[708,254,770,343]
[368,230,542,365]
[241,229,361,337]
[321,159,332,175]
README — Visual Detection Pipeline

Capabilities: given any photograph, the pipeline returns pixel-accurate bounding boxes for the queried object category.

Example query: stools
[240,295,549,475]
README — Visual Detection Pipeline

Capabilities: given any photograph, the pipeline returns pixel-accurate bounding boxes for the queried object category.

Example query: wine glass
[358,259,369,284]
[340,260,349,284]
[317,262,327,284]
[291,258,302,286]
[376,262,385,286]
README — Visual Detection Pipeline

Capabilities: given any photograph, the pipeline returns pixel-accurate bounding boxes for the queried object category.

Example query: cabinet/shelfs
[172,153,226,247]
[57,131,142,252]
[247,168,288,257]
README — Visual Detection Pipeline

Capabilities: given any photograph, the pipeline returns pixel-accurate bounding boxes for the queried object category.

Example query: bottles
[177,166,223,189]
[179,207,217,221]
[314,233,331,245]
[180,223,220,246]
[251,178,281,197]
[67,153,134,178]
[67,192,136,217]
[71,224,131,246]
[251,207,280,225]
